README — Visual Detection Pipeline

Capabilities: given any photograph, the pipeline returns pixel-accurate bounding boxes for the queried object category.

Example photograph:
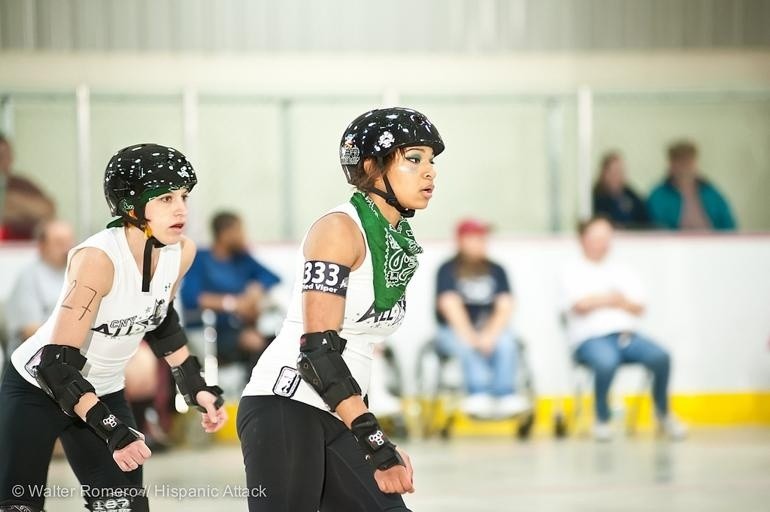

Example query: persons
[0,143,228,512]
[237,107,446,512]
[556,213,686,439]
[181,212,281,378]
[647,143,735,230]
[431,218,529,419]
[0,220,76,362]
[595,152,654,230]
[0,135,57,241]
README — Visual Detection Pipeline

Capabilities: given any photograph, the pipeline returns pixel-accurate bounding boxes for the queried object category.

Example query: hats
[453,220,493,236]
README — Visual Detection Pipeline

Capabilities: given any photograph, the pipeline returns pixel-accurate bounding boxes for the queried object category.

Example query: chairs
[2,300,671,442]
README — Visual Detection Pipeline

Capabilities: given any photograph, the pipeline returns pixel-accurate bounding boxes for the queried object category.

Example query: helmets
[102,142,198,218]
[337,106,446,187]
